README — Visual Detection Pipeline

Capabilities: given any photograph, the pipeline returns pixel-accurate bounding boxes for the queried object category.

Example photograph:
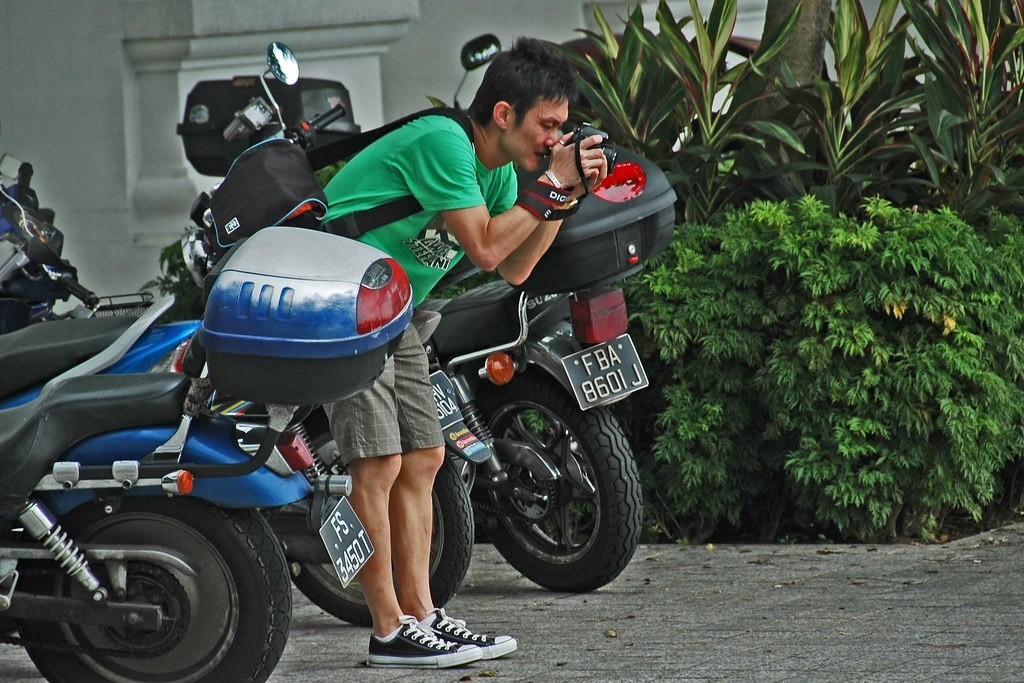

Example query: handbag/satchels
[202,138,328,259]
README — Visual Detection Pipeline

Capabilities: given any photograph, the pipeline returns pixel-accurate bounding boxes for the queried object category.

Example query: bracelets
[565,198,578,208]
[542,170,561,189]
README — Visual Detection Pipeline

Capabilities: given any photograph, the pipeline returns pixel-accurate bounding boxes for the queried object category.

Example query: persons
[320,36,609,670]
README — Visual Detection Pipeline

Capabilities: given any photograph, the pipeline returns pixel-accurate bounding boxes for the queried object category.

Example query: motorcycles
[1,223,414,682]
[0,36,678,630]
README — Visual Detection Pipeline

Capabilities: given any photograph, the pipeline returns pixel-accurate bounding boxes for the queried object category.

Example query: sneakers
[367,615,483,669]
[426,607,517,660]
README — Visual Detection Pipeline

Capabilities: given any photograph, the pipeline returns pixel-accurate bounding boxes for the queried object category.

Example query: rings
[558,140,565,145]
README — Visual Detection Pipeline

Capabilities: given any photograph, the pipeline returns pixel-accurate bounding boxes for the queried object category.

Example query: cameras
[565,121,618,174]
